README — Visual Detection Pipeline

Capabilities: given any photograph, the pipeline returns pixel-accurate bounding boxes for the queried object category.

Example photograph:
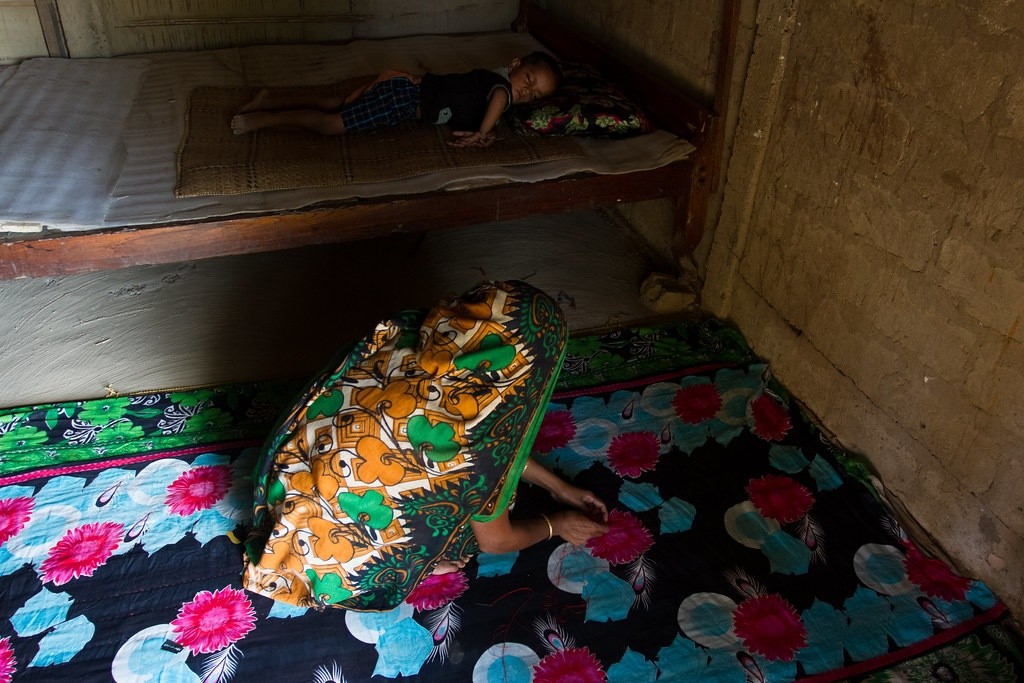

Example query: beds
[0,0,721,291]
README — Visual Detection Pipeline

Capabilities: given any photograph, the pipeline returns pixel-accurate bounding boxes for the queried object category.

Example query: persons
[241,279,627,603]
[231,50,562,148]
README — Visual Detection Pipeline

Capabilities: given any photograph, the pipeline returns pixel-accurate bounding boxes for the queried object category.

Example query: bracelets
[522,457,531,477]
[538,513,553,540]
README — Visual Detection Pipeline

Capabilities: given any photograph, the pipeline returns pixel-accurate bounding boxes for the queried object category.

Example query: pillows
[508,47,657,141]
